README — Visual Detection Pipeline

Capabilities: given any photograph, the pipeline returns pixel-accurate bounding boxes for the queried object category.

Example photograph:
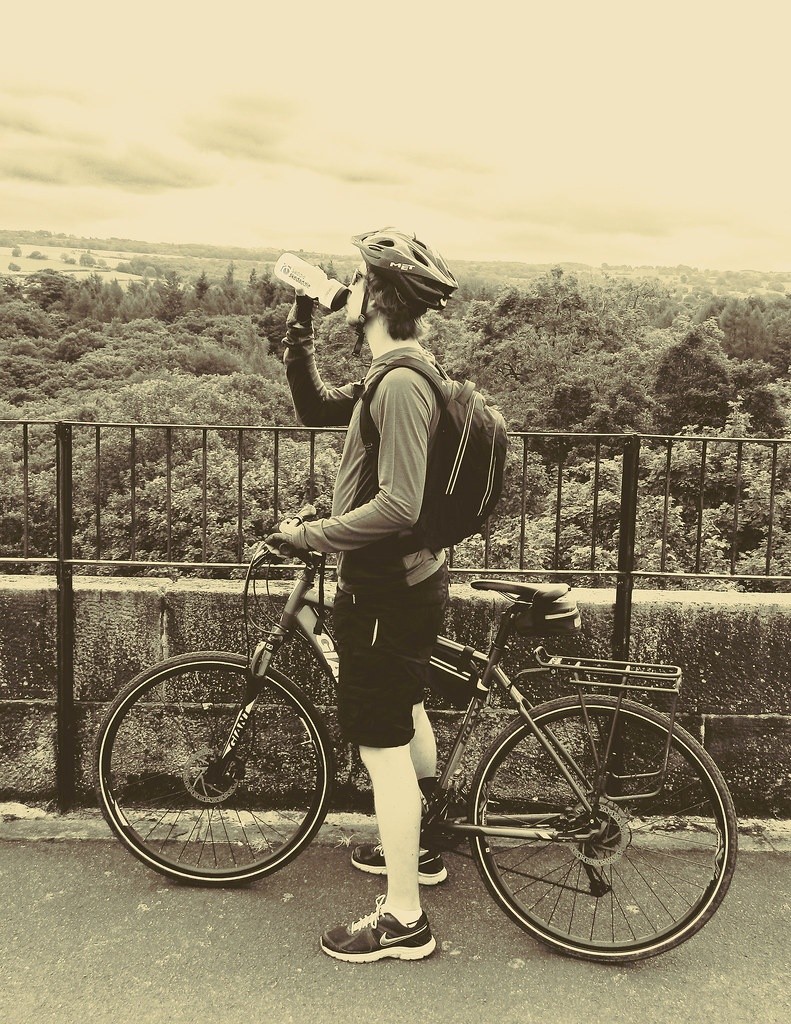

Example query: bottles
[273,252,351,312]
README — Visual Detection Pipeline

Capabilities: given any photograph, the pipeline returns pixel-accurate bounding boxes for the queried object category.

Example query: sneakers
[319,904,437,963]
[350,839,447,886]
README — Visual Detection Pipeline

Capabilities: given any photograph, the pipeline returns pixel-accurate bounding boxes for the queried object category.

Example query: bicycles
[91,501,738,964]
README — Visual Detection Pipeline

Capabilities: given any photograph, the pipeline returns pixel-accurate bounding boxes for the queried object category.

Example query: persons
[274,223,463,965]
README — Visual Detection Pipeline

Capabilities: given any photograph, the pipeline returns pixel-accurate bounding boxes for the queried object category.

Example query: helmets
[349,216,458,311]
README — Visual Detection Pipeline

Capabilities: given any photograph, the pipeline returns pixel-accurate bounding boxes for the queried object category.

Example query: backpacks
[359,356,508,551]
